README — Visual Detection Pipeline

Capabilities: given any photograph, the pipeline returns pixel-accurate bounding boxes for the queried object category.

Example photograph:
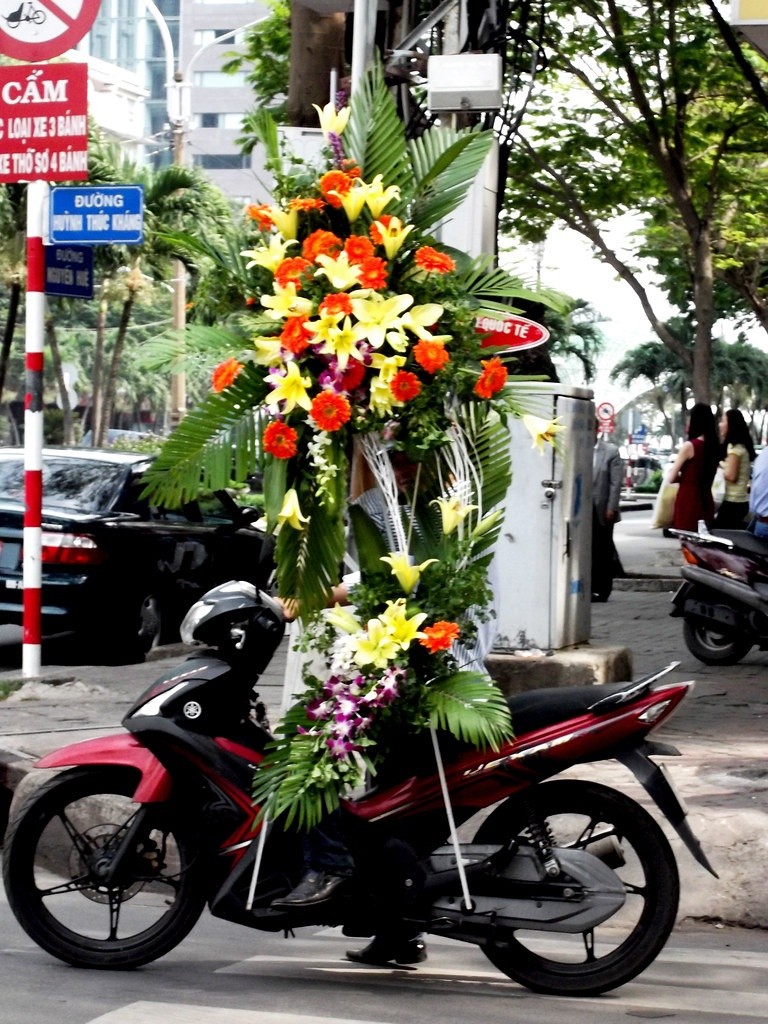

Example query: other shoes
[591,592,609,604]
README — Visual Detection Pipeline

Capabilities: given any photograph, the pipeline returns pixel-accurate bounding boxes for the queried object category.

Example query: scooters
[666,520,767,664]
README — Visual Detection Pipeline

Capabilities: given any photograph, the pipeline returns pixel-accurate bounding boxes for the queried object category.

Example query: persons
[277,455,498,966]
[592,421,627,599]
[746,446,768,534]
[713,408,751,532]
[667,404,719,567]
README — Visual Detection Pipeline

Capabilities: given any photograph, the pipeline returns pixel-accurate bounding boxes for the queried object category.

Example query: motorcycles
[0,567,724,997]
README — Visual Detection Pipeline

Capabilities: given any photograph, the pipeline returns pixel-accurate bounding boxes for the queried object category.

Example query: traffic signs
[46,183,143,301]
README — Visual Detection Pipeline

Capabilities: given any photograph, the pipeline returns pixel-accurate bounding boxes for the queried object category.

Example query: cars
[200,449,264,490]
[0,446,286,664]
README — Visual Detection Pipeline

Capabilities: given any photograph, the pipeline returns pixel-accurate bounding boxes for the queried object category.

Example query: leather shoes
[272,868,354,908]
[344,936,427,964]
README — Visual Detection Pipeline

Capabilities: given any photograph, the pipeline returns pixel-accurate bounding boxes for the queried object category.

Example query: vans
[620,459,662,490]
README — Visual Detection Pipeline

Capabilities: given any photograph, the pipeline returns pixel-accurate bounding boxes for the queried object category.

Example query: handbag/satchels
[711,466,727,508]
[650,479,681,531]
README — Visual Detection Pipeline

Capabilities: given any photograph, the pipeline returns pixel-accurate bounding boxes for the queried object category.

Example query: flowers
[140,69,568,828]
